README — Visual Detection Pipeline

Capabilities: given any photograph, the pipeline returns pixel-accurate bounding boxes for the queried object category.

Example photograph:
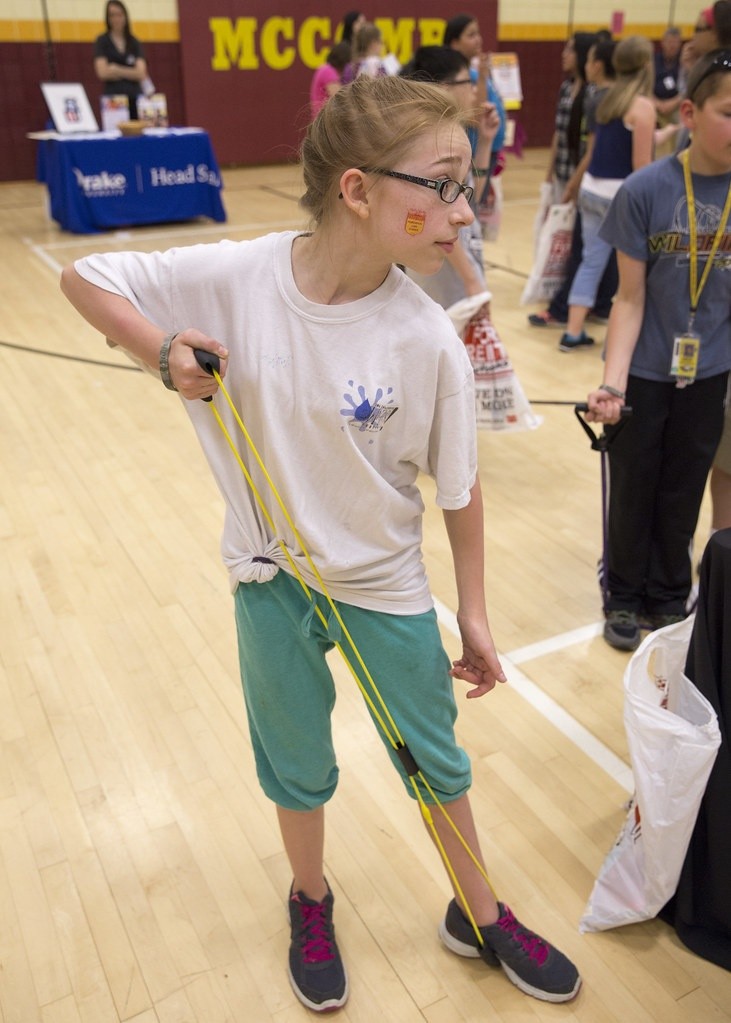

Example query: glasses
[436,75,478,91]
[689,48,731,102]
[338,165,474,208]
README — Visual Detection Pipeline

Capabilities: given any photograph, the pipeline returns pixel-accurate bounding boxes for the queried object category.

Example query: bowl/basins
[118,124,145,135]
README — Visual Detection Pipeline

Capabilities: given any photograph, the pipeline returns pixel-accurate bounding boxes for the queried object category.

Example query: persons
[585,42,731,652]
[398,43,500,315]
[526,0,731,542]
[685,345,693,356]
[57,61,582,1015]
[311,9,402,126]
[443,15,505,204]
[93,0,157,132]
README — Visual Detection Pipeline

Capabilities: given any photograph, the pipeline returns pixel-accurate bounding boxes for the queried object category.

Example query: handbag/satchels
[578,616,721,932]
[474,174,502,242]
[533,176,559,251]
[446,293,543,434]
[520,199,576,306]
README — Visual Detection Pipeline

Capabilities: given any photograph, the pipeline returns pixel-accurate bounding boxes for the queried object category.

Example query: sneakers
[288,873,348,1011]
[640,608,687,632]
[603,605,639,651]
[439,896,582,1003]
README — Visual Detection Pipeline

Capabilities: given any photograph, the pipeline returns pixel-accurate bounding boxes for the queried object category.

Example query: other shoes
[529,310,566,327]
[559,330,596,353]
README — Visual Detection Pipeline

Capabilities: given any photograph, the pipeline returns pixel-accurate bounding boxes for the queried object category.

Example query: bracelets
[472,164,493,177]
[599,385,626,399]
[160,331,178,391]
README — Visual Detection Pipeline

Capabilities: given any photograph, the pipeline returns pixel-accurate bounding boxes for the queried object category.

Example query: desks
[39,127,227,235]
